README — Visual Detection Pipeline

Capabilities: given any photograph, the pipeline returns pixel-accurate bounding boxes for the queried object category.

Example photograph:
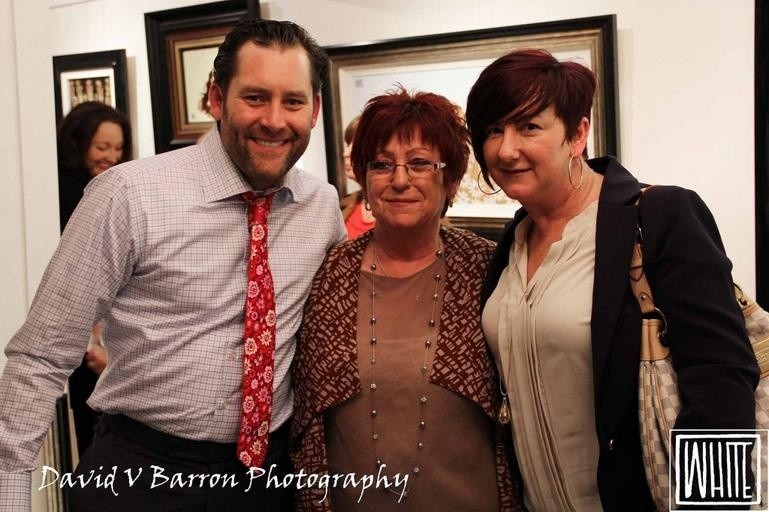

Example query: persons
[1,18,353,511]
[336,116,379,242]
[290,83,525,510]
[467,48,769,511]
[58,100,131,463]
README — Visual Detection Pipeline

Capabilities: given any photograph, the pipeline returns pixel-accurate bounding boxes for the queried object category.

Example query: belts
[103,412,238,465]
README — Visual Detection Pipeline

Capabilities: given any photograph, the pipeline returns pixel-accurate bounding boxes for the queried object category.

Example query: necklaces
[374,253,389,277]
[367,242,446,497]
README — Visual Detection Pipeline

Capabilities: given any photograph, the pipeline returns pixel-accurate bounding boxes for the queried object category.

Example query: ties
[234,191,278,481]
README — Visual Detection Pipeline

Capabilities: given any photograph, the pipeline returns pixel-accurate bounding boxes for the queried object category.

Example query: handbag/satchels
[630,183,768,512]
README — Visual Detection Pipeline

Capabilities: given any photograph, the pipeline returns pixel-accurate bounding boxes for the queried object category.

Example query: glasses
[365,158,447,180]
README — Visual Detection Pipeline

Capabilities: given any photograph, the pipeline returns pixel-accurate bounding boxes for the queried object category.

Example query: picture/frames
[143,0,264,156]
[316,11,622,242]
[52,47,128,126]
